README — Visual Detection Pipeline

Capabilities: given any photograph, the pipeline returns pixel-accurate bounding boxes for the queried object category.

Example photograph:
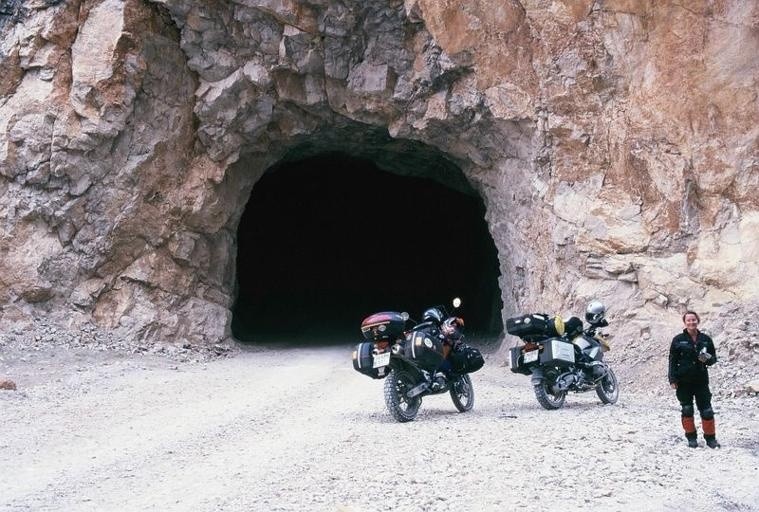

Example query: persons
[667,310,721,450]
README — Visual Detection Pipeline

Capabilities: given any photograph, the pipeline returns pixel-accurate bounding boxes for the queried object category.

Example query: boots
[703,433,721,449]
[685,430,699,448]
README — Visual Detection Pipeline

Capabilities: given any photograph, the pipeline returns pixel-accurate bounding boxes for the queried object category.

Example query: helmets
[422,307,445,327]
[584,300,606,326]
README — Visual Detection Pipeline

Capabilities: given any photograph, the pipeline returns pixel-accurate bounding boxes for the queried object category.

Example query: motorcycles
[353,296,619,422]
